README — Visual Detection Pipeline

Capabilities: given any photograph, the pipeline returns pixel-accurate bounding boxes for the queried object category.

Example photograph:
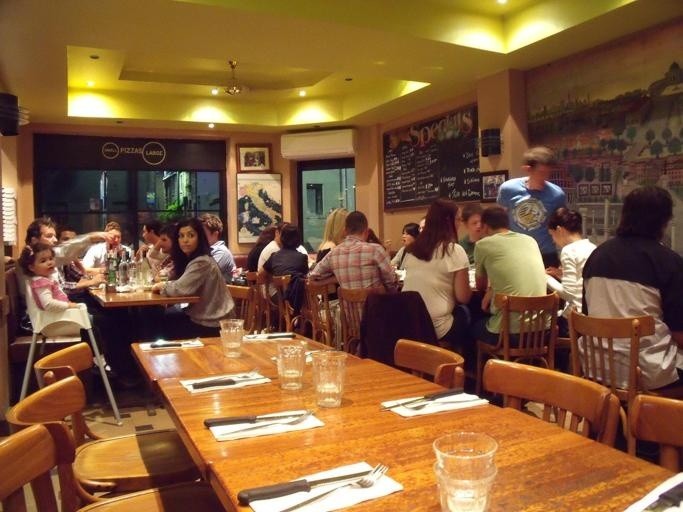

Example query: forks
[275,462,397,512]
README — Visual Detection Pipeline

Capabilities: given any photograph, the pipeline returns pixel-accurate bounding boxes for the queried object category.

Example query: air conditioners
[280,128,358,160]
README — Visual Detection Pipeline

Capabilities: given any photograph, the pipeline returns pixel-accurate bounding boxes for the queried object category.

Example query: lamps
[224,56,250,97]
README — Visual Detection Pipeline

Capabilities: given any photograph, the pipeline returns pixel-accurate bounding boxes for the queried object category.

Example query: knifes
[383,385,480,414]
[204,408,320,430]
[237,467,374,506]
[147,340,205,349]
[249,334,299,340]
[190,371,268,392]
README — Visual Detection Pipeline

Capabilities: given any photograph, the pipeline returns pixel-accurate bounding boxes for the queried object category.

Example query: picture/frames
[236,143,274,173]
[480,170,509,203]
[236,173,282,244]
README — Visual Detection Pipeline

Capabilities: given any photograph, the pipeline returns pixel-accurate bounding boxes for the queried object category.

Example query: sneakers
[114,374,144,390]
[92,356,111,372]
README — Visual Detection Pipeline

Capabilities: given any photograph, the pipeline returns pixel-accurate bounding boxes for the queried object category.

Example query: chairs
[0,246,683,511]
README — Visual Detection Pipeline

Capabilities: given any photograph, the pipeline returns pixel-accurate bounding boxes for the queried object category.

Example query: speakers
[0,93,20,136]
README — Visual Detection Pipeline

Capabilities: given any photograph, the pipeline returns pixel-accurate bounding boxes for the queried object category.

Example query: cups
[314,351,346,406]
[276,345,309,388]
[432,433,498,512]
[218,319,246,354]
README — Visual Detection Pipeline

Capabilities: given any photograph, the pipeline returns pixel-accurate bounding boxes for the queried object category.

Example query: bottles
[101,246,164,294]
